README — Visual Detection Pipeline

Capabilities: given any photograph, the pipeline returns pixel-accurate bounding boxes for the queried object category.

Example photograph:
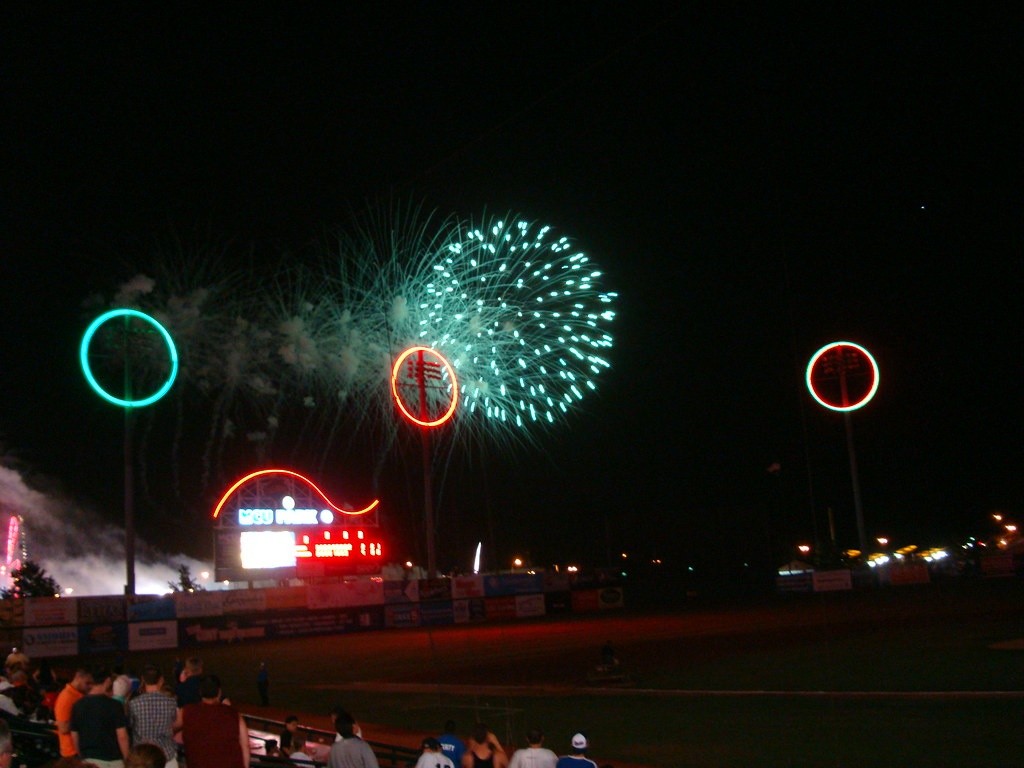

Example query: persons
[327,707,378,768]
[556,734,597,768]
[265,715,315,768]
[412,717,509,768]
[0,652,251,768]
[508,724,559,768]
[257,664,270,705]
[601,640,614,674]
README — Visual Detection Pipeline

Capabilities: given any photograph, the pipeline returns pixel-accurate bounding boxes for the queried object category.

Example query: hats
[423,737,437,749]
[572,733,587,749]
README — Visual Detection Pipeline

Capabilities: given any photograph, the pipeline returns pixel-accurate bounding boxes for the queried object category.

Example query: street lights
[391,345,459,581]
[77,302,185,599]
[805,338,887,558]
